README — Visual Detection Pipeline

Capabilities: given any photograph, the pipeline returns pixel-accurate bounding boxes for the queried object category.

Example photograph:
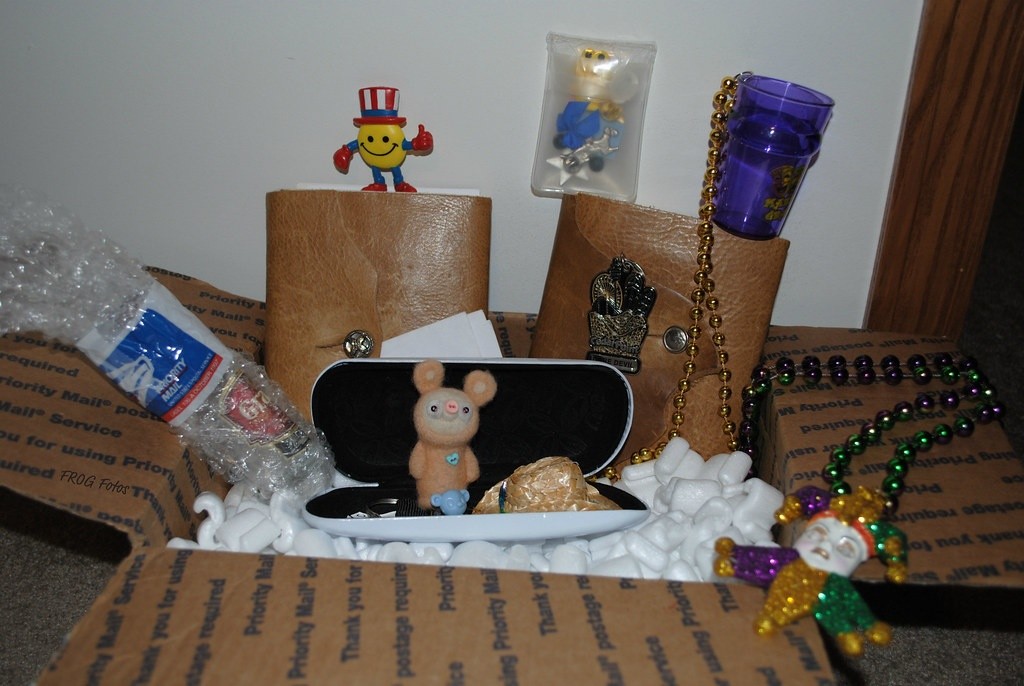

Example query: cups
[712,72,834,241]
